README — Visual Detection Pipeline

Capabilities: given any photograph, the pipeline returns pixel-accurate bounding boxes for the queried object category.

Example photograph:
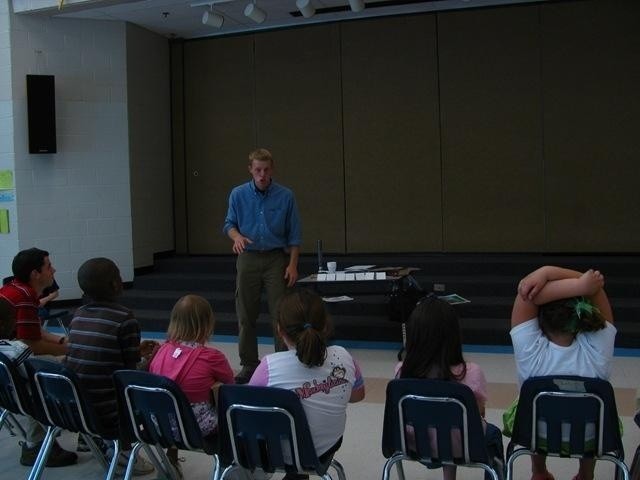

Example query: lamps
[349,0,365,14]
[244,4,266,25]
[201,10,225,29]
[295,0,315,19]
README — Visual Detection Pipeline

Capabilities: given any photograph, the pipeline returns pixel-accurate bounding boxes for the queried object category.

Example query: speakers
[26,74,57,154]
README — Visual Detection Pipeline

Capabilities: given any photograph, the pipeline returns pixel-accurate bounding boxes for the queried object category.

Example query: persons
[39,279,59,327]
[223,148,304,386]
[0,246,78,467]
[148,294,238,469]
[246,286,366,480]
[385,298,506,479]
[65,256,160,446]
[503,264,624,454]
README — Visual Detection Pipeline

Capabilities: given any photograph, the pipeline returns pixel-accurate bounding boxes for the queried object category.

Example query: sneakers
[19,437,78,468]
[234,360,261,383]
[76,433,107,453]
[106,448,154,475]
[160,457,184,479]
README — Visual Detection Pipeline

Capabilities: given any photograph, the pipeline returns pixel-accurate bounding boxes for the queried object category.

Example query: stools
[42,308,70,337]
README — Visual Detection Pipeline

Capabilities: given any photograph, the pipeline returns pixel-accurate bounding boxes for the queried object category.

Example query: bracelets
[58,337,64,344]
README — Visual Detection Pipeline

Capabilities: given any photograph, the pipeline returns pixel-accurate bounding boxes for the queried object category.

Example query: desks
[297,264,423,351]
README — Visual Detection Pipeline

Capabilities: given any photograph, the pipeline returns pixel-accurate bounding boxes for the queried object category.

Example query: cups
[327,262,336,274]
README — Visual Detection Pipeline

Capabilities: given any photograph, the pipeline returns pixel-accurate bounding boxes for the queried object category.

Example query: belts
[242,248,283,254]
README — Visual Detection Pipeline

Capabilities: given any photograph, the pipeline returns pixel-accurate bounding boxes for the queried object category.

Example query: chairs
[113,370,240,480]
[505,375,630,479]
[33,371,168,480]
[0,407,27,442]
[0,352,108,480]
[381,379,501,479]
[219,384,346,480]
